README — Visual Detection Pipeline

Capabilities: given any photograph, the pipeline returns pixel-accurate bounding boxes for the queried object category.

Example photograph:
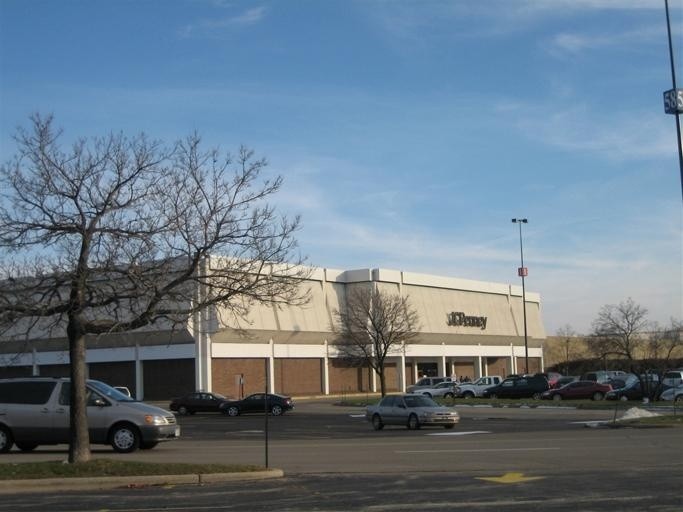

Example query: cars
[169,392,226,414]
[366,394,460,430]
[219,393,295,417]
[407,370,683,402]
[113,387,130,398]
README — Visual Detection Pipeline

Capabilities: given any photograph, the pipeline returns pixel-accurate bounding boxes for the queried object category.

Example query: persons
[460,375,472,382]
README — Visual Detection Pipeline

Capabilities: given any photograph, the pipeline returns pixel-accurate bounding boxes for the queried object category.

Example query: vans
[0,377,181,453]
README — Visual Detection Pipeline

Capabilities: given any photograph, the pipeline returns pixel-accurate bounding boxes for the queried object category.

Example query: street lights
[512,218,529,374]
